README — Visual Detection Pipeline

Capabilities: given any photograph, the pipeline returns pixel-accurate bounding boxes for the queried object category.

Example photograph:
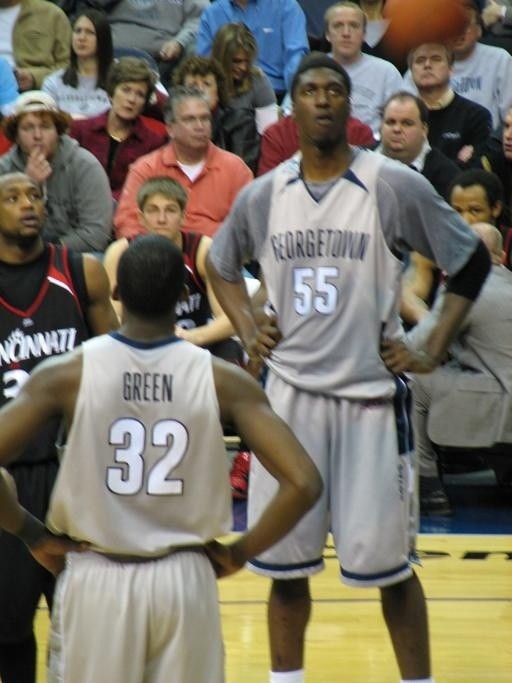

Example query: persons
[403,222,512,513]
[0,170,121,677]
[469,0,512,53]
[68,56,170,200]
[0,55,22,108]
[404,4,512,126]
[205,49,492,680]
[257,113,377,181]
[0,89,113,266]
[40,9,115,122]
[0,0,74,90]
[396,170,512,325]
[173,55,261,179]
[0,234,325,679]
[280,0,404,144]
[195,0,310,105]
[312,0,406,74]
[469,104,511,270]
[370,91,462,195]
[101,177,252,501]
[210,22,281,108]
[75,0,206,73]
[115,84,257,244]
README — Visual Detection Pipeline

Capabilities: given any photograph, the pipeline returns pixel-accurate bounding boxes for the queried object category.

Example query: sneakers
[231,451,249,499]
[418,491,455,518]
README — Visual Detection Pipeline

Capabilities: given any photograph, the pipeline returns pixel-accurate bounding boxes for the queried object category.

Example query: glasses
[171,115,209,126]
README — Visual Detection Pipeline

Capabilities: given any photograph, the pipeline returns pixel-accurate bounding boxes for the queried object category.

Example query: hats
[14,89,57,113]
